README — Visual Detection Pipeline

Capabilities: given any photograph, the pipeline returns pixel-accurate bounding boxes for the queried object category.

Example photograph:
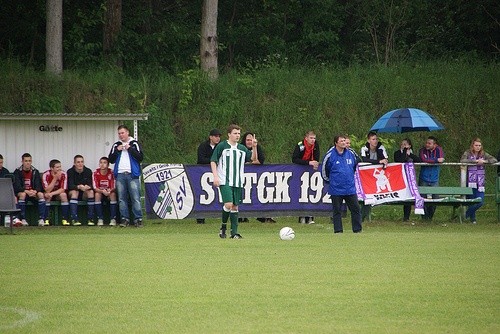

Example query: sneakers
[230,234,242,239]
[219,227,227,238]
[12,215,144,227]
[471,217,476,224]
[299,219,316,224]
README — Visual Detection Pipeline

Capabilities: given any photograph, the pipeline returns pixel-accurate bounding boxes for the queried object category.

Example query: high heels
[421,217,433,222]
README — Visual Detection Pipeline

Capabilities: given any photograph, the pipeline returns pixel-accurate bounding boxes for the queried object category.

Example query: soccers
[279,227,295,240]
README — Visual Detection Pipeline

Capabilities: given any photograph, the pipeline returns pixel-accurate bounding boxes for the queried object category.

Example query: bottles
[383,156,388,168]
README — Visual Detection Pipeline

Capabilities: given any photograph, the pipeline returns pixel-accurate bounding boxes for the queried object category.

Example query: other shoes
[238,217,277,224]
[361,209,370,222]
[403,216,410,221]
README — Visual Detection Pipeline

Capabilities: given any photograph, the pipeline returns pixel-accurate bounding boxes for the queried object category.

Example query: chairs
[0,178,21,234]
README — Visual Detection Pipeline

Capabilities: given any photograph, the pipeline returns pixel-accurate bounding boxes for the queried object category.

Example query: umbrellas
[369,107,446,137]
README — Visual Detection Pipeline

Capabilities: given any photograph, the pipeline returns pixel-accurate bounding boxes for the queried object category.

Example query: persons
[210,124,259,239]
[196,130,225,224]
[66,155,97,226]
[237,133,266,222]
[91,157,117,226]
[419,135,445,221]
[107,125,144,228]
[393,137,420,223]
[11,153,46,226]
[291,130,320,224]
[459,136,498,223]
[322,130,389,234]
[0,154,20,227]
[42,159,70,225]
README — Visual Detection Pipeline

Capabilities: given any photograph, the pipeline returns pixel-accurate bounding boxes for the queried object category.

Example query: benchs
[0,201,118,225]
[367,186,482,225]
